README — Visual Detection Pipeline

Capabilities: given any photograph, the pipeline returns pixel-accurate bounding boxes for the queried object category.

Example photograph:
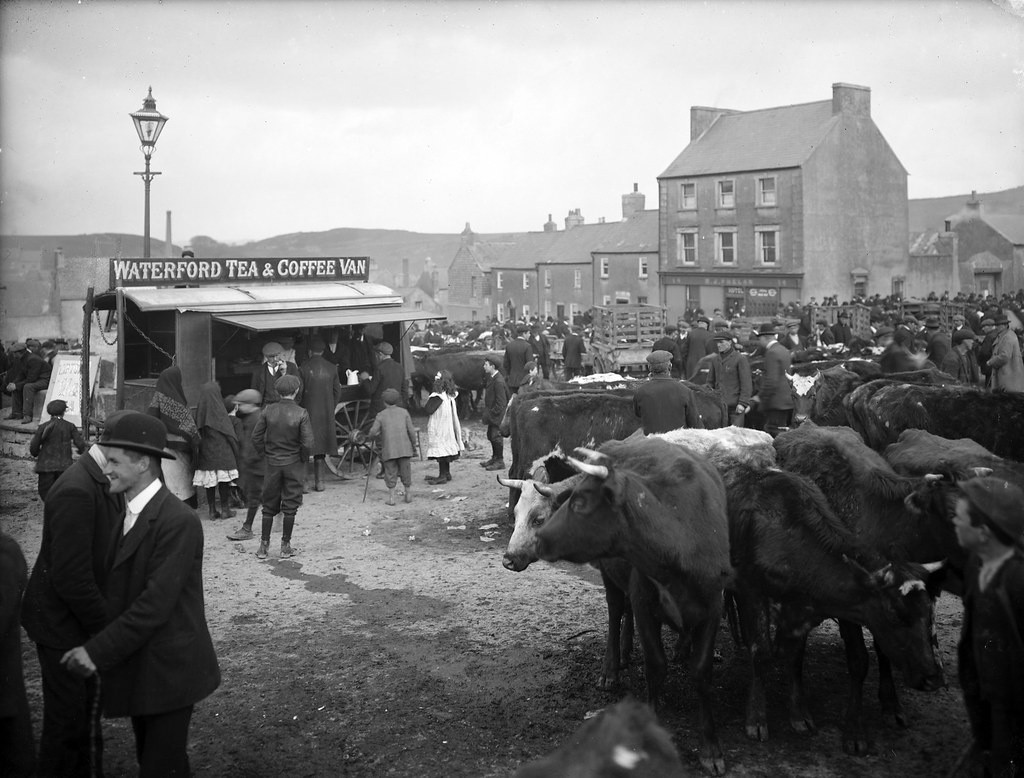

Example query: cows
[785,363,863,428]
[509,394,647,525]
[505,700,688,778]
[410,354,506,420]
[496,425,776,692]
[881,428,1024,502]
[705,456,948,762]
[497,380,644,437]
[671,378,728,429]
[773,424,965,684]
[789,339,965,387]
[410,347,484,407]
[841,379,1024,464]
[532,439,729,775]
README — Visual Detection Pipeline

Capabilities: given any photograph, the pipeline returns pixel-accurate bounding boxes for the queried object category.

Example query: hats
[374,342,394,355]
[563,316,569,320]
[382,388,400,404]
[571,325,580,331]
[517,325,533,332]
[957,477,1024,547]
[231,337,326,404]
[646,300,1010,365]
[5,337,68,352]
[47,400,68,415]
[95,409,177,461]
[486,355,502,368]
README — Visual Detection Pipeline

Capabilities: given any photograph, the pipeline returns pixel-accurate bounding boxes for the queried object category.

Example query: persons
[0,401,227,778]
[420,290,1024,434]
[924,474,1023,777]
[149,326,510,560]
[0,337,81,425]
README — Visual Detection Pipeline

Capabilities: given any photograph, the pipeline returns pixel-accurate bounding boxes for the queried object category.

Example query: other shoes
[22,415,32,424]
[208,502,298,559]
[481,457,505,471]
[375,474,452,506]
[4,413,23,420]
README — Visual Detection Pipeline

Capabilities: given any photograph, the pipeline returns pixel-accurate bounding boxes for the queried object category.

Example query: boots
[314,459,325,491]
[303,461,311,493]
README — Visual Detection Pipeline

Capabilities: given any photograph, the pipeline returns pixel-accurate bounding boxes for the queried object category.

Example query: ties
[122,506,131,534]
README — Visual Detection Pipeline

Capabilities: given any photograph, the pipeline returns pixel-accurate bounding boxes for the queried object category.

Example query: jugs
[345,369,360,386]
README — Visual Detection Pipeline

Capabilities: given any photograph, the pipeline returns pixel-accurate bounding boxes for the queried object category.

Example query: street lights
[129,85,169,259]
